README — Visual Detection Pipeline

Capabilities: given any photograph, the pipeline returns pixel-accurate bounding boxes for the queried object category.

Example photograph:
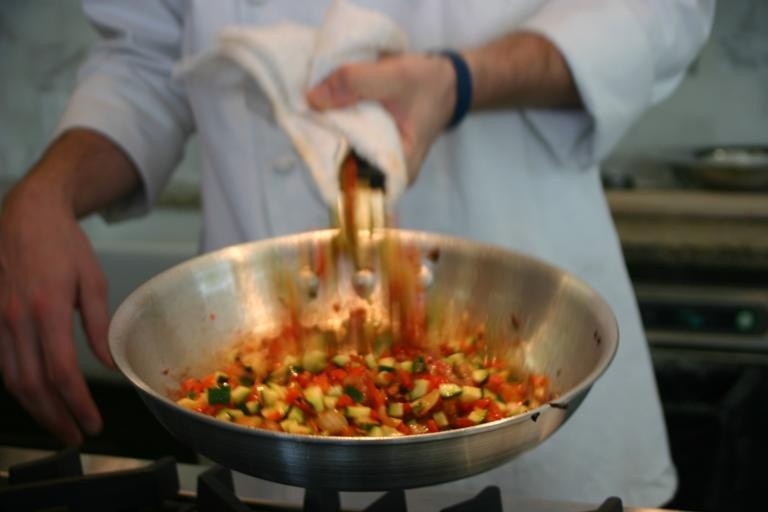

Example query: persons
[0,0,716,512]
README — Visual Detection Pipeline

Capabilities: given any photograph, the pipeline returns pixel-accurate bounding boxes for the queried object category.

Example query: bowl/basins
[673,144,768,192]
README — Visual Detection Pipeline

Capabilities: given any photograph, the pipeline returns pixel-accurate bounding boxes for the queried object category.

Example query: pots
[108,149,617,492]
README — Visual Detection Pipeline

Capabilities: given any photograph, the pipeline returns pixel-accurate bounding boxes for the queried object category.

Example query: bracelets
[439,47,473,130]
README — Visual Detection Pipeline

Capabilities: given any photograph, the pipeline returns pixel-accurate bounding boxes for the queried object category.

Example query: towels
[169,1,412,213]
